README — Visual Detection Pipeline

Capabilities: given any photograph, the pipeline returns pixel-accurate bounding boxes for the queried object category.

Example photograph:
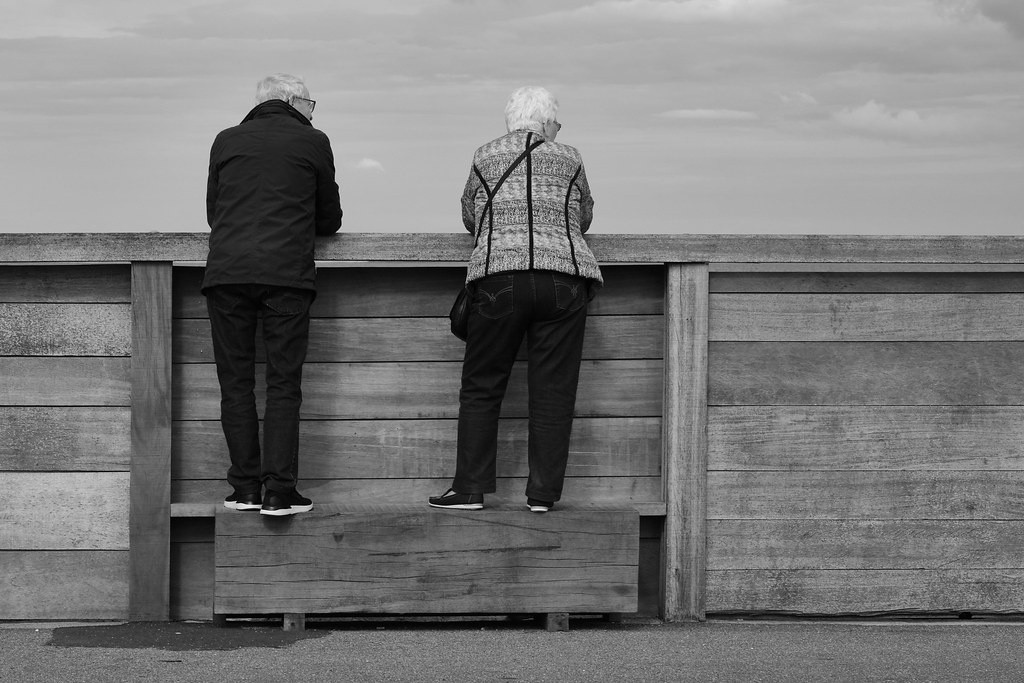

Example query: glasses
[553,121,561,131]
[296,96,316,113]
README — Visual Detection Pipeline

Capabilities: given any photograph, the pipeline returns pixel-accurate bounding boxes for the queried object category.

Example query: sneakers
[526,497,554,511]
[224,490,263,510]
[428,488,484,510]
[260,490,313,516]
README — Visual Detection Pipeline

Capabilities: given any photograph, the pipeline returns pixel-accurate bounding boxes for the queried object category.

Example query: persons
[201,72,342,517]
[428,85,604,517]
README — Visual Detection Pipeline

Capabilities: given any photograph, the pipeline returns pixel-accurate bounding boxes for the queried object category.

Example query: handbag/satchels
[450,288,474,342]
